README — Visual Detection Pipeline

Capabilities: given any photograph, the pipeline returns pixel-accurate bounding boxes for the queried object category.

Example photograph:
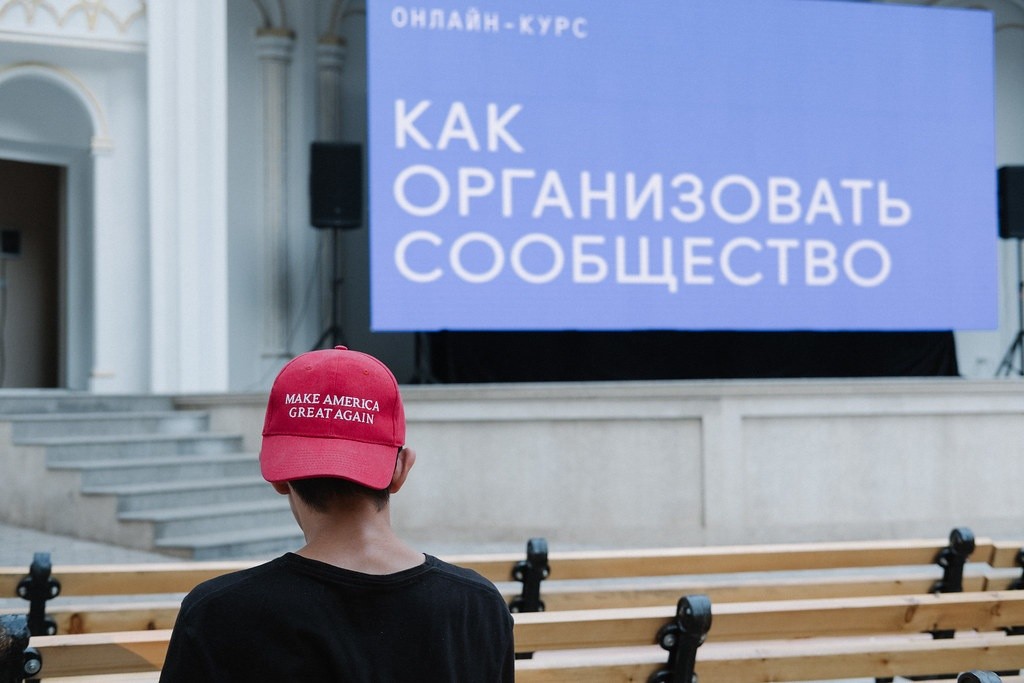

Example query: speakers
[997,165,1024,239]
[309,141,364,228]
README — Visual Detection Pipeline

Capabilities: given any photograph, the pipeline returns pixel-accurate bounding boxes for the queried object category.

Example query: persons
[158,346,517,683]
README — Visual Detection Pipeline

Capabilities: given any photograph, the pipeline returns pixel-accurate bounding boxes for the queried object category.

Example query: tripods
[308,228,349,355]
[995,239,1024,377]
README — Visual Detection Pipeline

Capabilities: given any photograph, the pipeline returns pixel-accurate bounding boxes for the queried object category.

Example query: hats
[260,346,406,491]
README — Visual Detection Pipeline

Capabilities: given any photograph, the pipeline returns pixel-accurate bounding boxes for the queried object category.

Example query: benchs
[0,527,1024,683]
